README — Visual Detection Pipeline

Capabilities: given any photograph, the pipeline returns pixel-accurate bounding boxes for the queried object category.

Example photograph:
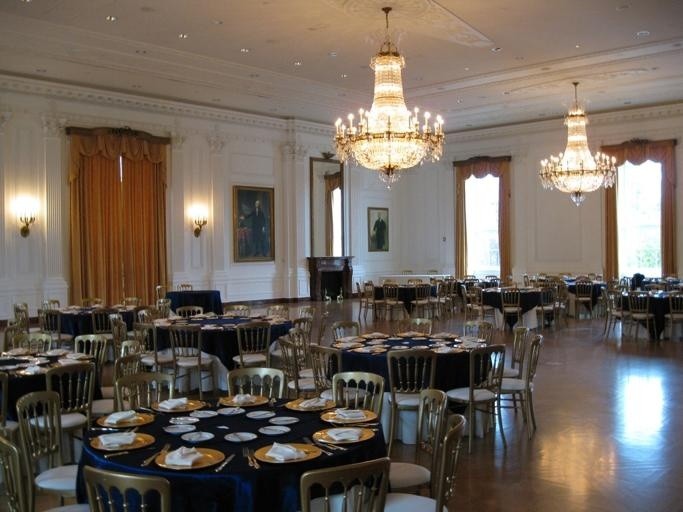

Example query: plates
[164,407,302,445]
[254,442,322,465]
[0,348,93,376]
[286,397,339,412]
[151,398,205,414]
[312,426,376,445]
[319,407,379,425]
[151,311,290,331]
[96,412,155,428]
[333,329,487,357]
[154,447,226,471]
[219,394,270,406]
[55,303,137,315]
[90,431,156,452]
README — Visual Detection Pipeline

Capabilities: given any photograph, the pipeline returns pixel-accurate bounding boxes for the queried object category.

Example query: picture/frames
[230,183,276,263]
[366,207,390,253]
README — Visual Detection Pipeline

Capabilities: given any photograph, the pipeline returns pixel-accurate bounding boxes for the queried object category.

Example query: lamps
[331,5,444,191]
[17,196,36,237]
[538,79,617,208]
[189,202,207,237]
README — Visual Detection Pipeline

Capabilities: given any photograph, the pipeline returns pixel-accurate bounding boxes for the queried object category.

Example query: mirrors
[308,151,345,259]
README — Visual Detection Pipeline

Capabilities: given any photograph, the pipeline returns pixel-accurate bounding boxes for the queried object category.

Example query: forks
[304,438,347,457]
[249,449,261,469]
[141,444,170,466]
[242,447,253,469]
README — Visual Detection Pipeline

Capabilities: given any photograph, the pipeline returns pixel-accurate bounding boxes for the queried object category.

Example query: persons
[239,200,268,257]
[374,212,386,250]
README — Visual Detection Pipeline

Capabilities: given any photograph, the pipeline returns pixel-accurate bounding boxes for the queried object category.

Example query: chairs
[1,269,568,512]
[567,273,682,350]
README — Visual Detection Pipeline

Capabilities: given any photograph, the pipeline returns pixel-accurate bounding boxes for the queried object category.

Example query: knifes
[216,402,220,406]
[90,427,129,431]
[139,406,164,415]
[214,453,236,474]
[103,450,141,458]
